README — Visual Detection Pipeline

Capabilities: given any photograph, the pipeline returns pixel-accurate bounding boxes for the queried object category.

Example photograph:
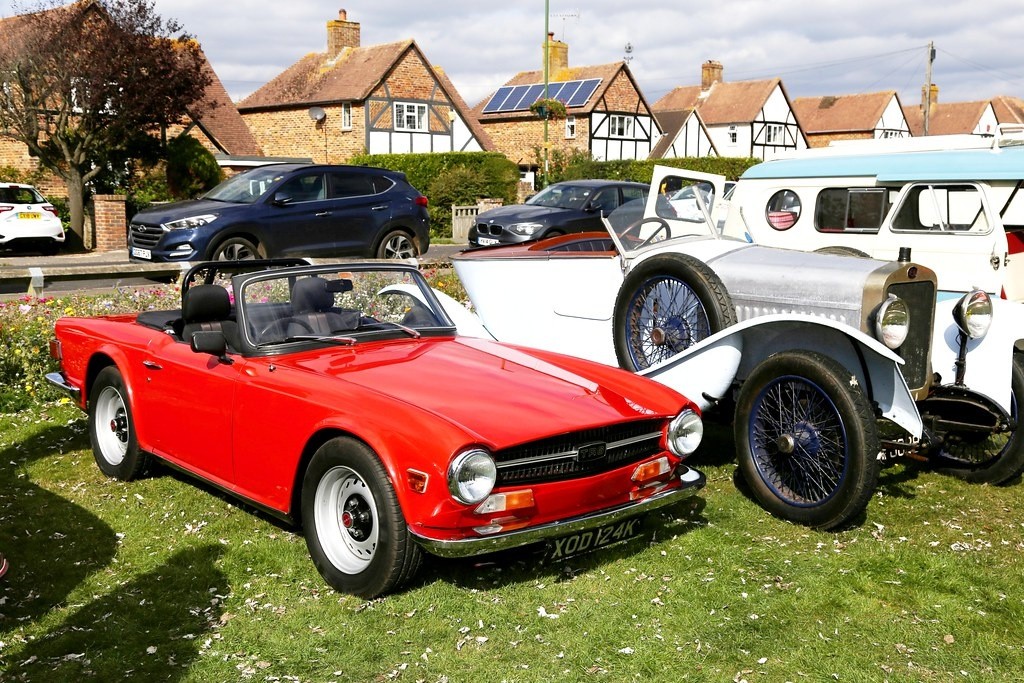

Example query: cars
[0,183,69,255]
[378,122,1024,528]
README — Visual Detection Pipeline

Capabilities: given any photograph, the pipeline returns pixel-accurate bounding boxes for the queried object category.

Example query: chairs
[274,178,325,202]
[289,276,350,338]
[181,285,243,353]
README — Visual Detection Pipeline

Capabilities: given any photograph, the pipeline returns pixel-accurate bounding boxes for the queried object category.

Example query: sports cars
[41,260,706,601]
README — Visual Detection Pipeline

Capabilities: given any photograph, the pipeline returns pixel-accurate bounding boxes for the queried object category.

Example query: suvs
[466,177,660,247]
[128,163,433,283]
